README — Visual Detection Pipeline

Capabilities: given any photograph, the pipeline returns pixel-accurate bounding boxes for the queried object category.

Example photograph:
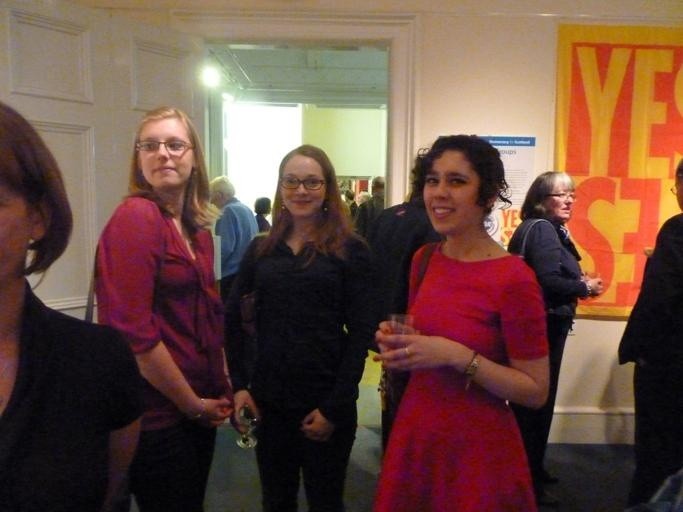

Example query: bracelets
[187,397,207,421]
[581,280,593,298]
[461,351,482,379]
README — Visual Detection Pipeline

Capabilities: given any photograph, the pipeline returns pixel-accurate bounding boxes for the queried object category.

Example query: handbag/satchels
[518,218,577,315]
[375,242,437,414]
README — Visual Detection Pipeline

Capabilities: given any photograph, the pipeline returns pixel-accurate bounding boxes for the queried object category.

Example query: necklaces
[173,216,194,254]
[444,238,498,258]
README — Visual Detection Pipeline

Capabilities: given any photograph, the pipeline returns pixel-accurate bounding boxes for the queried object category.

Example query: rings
[405,348,410,358]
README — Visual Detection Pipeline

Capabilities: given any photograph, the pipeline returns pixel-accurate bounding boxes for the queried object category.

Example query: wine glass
[236,405,261,451]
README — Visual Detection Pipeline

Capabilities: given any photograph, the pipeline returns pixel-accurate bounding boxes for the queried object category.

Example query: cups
[586,271,601,298]
[387,313,416,378]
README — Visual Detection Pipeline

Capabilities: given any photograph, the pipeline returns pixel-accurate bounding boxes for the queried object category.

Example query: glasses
[280,176,325,191]
[671,183,680,194]
[547,193,576,199]
[135,140,195,156]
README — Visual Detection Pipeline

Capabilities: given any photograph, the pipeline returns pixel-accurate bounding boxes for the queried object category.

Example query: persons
[357,191,370,206]
[254,197,272,232]
[509,171,604,498]
[206,176,258,428]
[617,159,683,511]
[1,101,146,512]
[93,102,236,512]
[353,176,385,232]
[345,189,358,221]
[371,134,551,512]
[223,145,379,512]
[367,188,447,465]
